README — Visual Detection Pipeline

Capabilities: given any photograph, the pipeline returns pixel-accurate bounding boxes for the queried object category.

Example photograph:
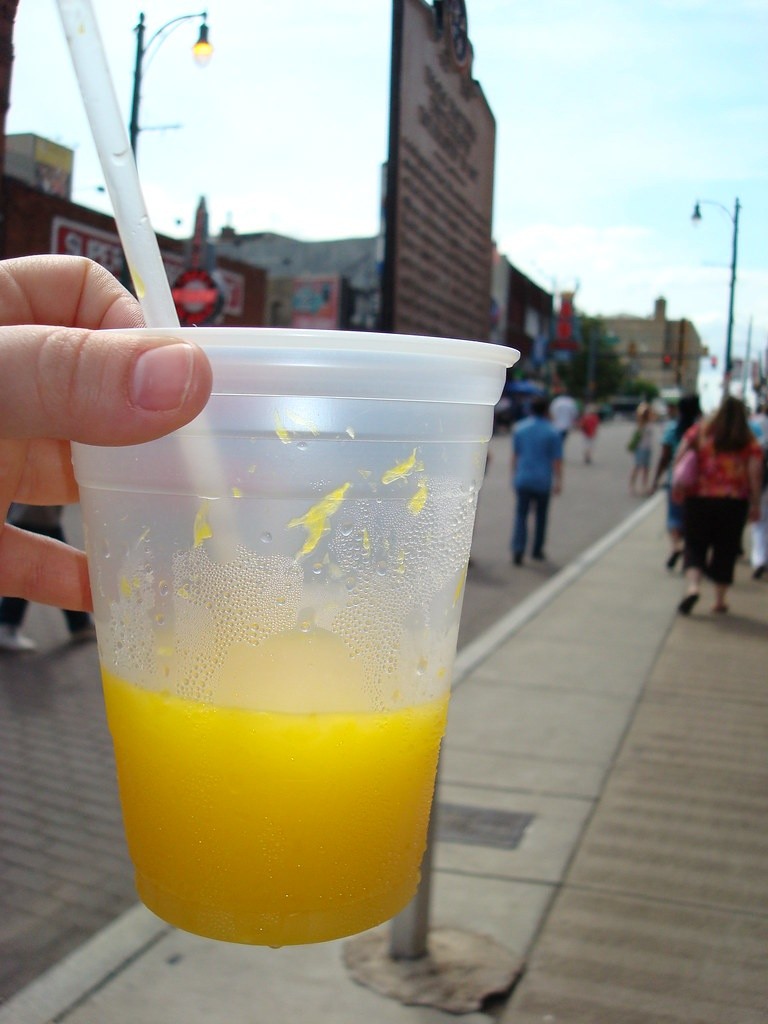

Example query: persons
[0,496,100,651]
[0,252,212,617]
[473,372,768,618]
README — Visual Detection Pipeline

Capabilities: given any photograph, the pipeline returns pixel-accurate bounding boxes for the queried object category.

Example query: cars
[498,381,550,427]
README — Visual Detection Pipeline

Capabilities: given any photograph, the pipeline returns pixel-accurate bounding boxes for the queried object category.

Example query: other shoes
[532,551,545,559]
[666,549,683,569]
[752,564,766,579]
[512,550,523,564]
[712,603,729,613]
[679,593,697,613]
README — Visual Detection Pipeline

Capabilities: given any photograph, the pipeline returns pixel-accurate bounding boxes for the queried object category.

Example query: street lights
[692,195,741,406]
[128,11,212,166]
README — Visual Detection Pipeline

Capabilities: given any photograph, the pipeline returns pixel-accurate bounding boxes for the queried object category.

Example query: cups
[70,327,520,947]
